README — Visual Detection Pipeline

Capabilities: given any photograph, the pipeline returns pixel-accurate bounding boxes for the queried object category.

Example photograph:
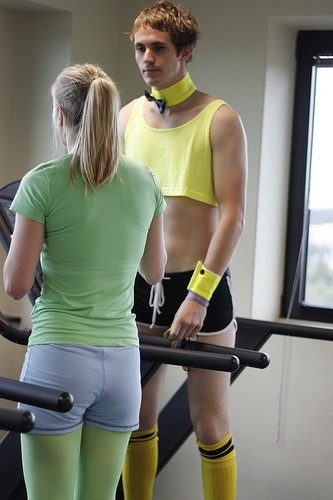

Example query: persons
[119,2,253,500]
[3,64,168,500]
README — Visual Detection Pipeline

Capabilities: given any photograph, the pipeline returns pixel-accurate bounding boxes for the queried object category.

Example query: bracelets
[186,291,209,307]
